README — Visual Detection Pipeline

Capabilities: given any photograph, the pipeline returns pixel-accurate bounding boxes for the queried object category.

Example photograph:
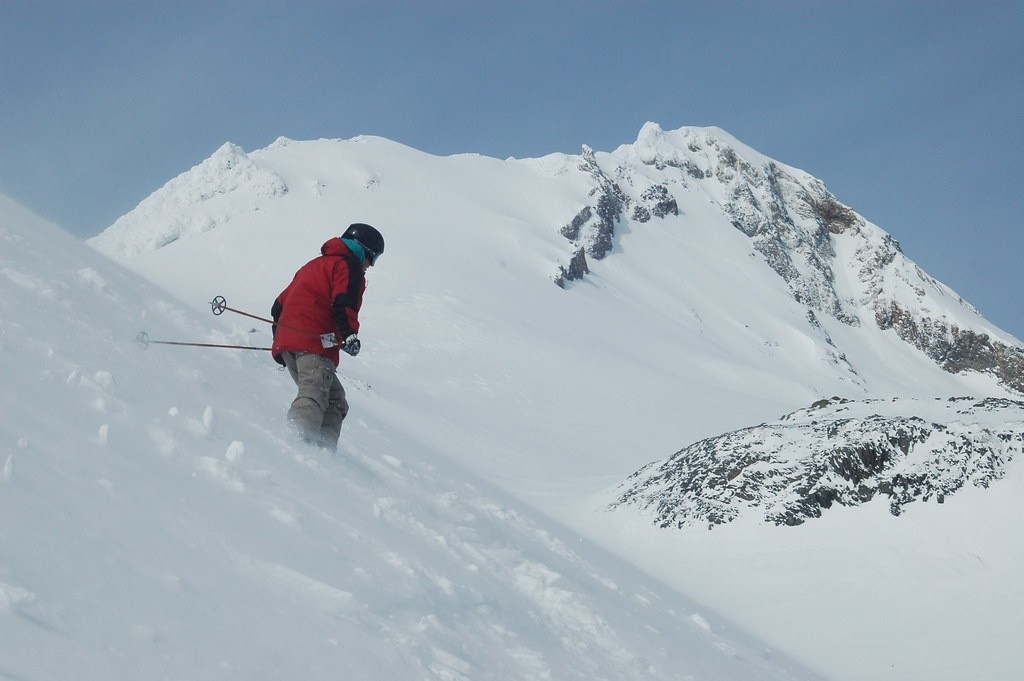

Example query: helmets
[341,223,385,265]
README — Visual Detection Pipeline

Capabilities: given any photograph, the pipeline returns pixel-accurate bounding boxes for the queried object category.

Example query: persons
[270,223,385,457]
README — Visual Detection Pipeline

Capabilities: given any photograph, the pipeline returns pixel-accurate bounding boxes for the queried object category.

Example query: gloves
[345,333,360,357]
